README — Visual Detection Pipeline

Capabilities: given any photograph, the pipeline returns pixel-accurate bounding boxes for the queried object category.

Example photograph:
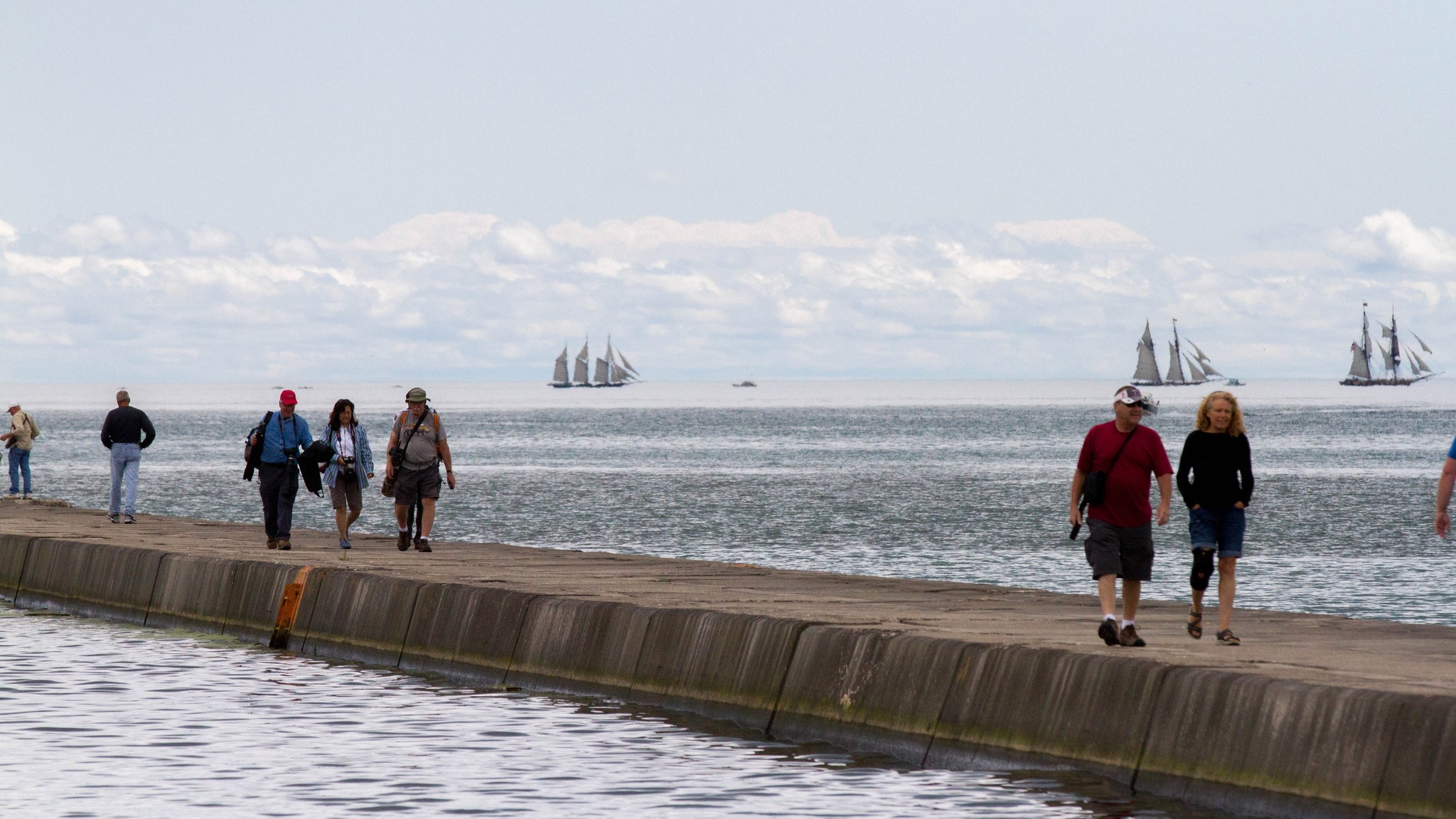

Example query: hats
[280,389,298,405]
[6,400,20,413]
[1112,389,1149,406]
[405,388,431,403]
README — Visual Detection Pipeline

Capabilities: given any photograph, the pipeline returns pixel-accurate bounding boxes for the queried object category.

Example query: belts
[115,442,139,444]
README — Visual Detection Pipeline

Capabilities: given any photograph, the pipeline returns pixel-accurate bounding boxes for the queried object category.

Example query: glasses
[1125,401,1144,408]
[338,399,350,402]
[411,401,423,406]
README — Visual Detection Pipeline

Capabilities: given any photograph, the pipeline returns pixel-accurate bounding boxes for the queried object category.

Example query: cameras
[343,456,356,482]
[284,447,297,468]
[5,437,17,448]
[381,447,406,498]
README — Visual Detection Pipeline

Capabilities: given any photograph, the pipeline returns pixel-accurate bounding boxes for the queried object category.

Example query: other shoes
[4,493,21,499]
[341,539,352,549]
[339,528,349,545]
[277,540,292,549]
[266,537,277,548]
[408,527,413,547]
[414,533,421,549]
[24,492,32,499]
[419,539,433,553]
[397,529,409,551]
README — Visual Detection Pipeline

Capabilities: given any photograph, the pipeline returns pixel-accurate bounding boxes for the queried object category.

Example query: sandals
[1217,629,1242,646]
[1187,605,1202,639]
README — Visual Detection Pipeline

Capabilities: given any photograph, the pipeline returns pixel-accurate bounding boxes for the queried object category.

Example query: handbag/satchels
[245,411,274,469]
[1083,432,1133,506]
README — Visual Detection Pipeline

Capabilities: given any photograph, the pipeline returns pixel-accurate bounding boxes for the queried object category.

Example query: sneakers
[1097,618,1120,645]
[1121,624,1147,647]
[124,515,137,523]
[107,512,120,524]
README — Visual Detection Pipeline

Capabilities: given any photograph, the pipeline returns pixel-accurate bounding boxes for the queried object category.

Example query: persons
[1177,389,1254,646]
[322,399,374,548]
[385,387,456,552]
[0,401,40,500]
[250,390,314,549]
[1435,435,1456,540]
[100,391,156,524]
[1070,386,1172,648]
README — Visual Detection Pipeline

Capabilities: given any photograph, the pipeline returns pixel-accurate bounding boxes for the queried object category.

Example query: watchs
[447,469,453,473]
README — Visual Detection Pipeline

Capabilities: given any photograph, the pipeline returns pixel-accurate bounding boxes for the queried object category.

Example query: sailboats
[1338,301,1442,386]
[1131,317,1227,386]
[547,334,639,388]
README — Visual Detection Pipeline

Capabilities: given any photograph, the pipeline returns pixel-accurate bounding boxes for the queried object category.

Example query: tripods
[243,421,267,482]
